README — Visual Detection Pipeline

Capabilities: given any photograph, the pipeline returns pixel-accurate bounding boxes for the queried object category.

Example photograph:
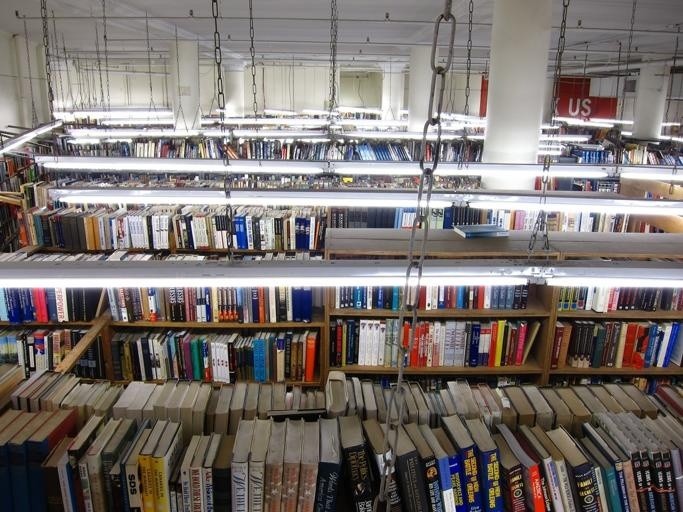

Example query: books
[1,107,681,512]
